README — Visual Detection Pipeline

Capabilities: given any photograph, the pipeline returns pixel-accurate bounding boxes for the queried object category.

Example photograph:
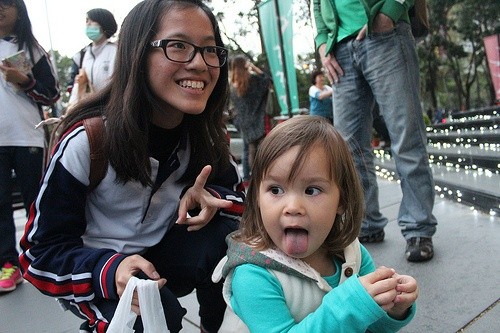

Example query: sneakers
[360,230,384,243]
[0,263,23,293]
[405,237,433,262]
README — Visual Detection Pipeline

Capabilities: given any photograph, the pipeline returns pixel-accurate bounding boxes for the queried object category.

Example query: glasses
[148,37,229,69]
[0,0,18,9]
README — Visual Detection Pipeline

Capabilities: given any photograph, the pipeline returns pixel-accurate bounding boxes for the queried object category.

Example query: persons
[212,114,418,333]
[432,103,445,125]
[226,54,273,195]
[18,0,248,333]
[310,0,438,262]
[0,0,61,292]
[65,9,118,117]
[308,70,333,125]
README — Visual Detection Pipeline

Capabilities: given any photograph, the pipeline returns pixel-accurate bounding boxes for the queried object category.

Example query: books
[2,50,38,95]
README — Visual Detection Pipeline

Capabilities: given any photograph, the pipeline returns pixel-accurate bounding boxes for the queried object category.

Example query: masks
[86,25,105,40]
